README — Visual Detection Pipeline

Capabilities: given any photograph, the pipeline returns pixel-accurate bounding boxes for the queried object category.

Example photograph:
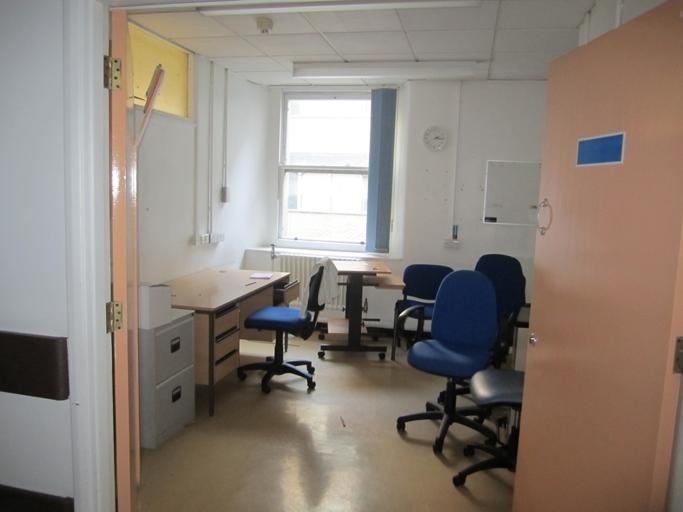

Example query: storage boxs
[139,282,171,328]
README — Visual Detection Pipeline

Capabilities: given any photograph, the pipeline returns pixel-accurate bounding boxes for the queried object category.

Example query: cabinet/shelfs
[138,307,198,452]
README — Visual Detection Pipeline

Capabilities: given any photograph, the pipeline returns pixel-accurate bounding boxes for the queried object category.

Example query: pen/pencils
[245,281,257,286]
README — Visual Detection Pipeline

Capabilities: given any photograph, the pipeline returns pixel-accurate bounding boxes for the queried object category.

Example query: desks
[318,260,407,362]
[165,266,302,417]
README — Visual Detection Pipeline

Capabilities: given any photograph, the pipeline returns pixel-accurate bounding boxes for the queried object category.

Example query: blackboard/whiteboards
[483,160,540,228]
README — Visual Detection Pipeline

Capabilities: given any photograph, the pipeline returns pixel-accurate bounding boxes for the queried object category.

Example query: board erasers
[485,216,497,222]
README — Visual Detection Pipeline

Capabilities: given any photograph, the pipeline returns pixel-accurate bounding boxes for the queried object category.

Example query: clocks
[423,126,447,151]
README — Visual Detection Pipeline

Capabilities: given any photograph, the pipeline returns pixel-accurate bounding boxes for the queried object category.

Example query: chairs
[391,253,527,490]
[236,266,324,392]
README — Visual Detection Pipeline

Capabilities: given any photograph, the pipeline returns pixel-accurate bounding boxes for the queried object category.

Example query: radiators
[271,253,353,312]
